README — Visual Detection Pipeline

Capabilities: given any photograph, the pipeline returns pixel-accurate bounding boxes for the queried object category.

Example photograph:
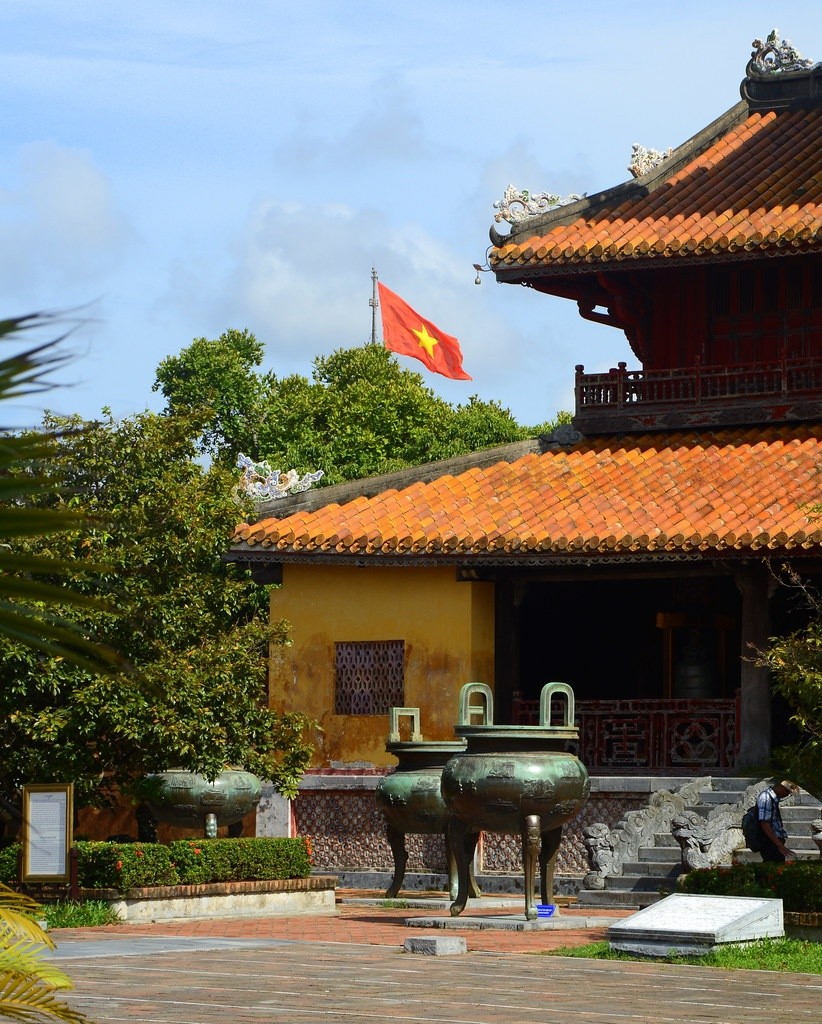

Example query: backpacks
[742,790,773,852]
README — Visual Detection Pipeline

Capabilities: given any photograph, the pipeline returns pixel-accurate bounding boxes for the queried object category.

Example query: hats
[780,780,797,795]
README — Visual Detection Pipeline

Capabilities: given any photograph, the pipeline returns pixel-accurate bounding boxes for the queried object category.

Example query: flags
[378,281,473,380]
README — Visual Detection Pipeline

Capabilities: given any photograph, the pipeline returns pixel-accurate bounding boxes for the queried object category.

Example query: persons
[756,780,796,864]
